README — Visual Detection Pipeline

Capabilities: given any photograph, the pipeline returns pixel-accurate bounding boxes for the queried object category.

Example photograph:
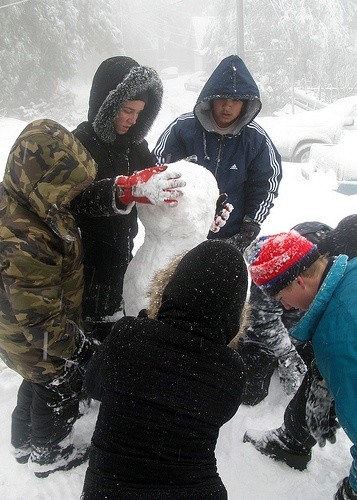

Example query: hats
[245,232,319,297]
[290,222,335,255]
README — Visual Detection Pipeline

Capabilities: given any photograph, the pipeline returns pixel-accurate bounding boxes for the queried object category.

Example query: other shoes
[76,395,90,420]
[13,443,89,478]
[243,425,312,472]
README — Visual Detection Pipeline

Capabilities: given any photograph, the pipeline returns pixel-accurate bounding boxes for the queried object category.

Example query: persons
[244,214,357,500]
[152,55,308,396]
[83,240,251,500]
[0,118,101,478]
[71,57,234,322]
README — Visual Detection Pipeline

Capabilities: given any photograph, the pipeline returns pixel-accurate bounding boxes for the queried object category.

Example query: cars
[266,96,357,190]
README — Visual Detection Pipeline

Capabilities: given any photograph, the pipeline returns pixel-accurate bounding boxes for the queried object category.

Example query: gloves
[227,218,261,253]
[305,380,341,447]
[239,328,267,353]
[278,354,308,396]
[65,329,102,373]
[115,165,187,208]
[335,477,357,500]
[210,192,234,233]
